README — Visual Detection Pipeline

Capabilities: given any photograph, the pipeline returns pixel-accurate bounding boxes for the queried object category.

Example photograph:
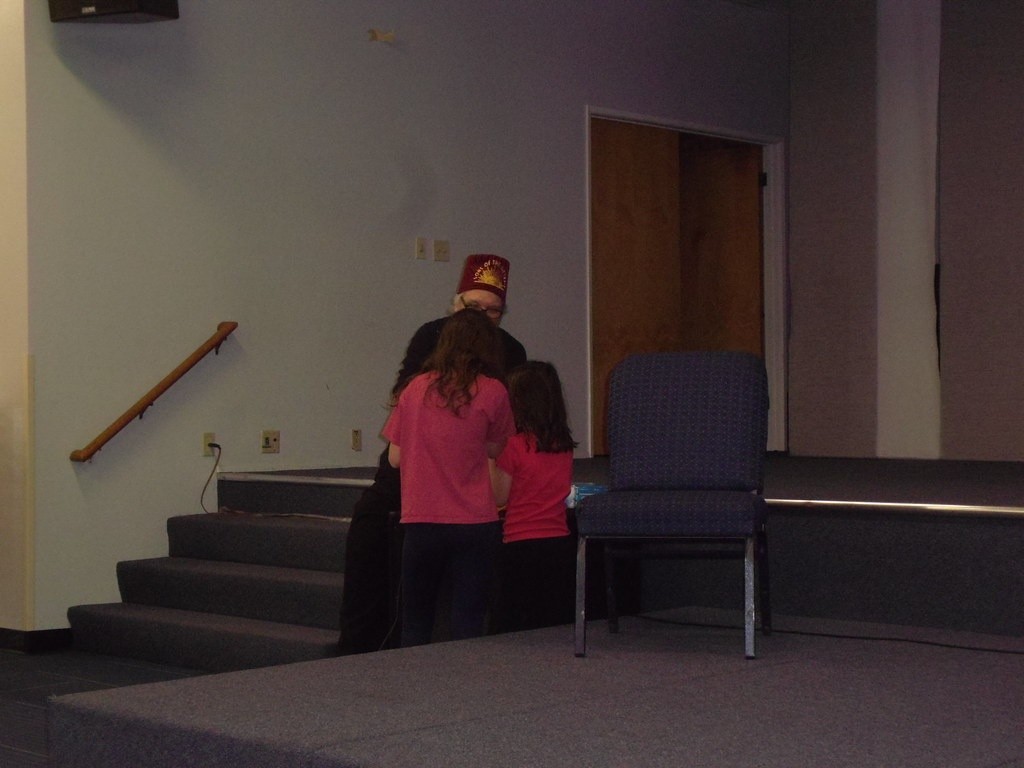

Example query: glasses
[461,297,502,320]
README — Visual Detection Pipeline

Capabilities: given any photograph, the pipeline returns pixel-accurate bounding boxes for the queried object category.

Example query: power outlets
[202,432,215,457]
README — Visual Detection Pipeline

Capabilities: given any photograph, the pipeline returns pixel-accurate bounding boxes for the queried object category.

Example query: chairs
[569,352,772,661]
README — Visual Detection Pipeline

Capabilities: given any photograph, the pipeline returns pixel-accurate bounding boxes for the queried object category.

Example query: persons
[339,254,526,653]
[382,306,515,648]
[487,359,578,634]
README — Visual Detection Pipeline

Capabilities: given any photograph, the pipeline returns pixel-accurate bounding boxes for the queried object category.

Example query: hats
[457,253,510,304]
